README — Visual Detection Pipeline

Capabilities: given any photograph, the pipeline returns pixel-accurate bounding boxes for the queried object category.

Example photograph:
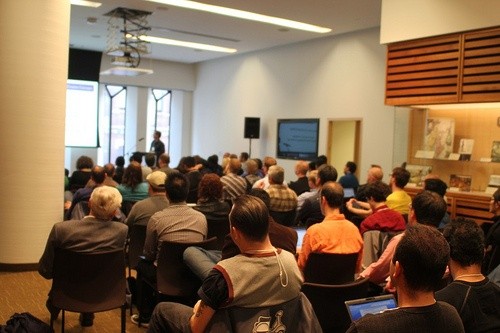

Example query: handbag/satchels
[1,312,54,333]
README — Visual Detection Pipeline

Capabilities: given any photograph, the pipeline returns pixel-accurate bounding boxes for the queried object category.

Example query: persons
[434,216,500,333]
[64,151,499,295]
[146,195,304,333]
[38,186,129,327]
[150,130,165,167]
[346,226,465,333]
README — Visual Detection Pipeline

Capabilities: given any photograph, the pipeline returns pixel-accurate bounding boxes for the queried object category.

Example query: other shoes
[127,276,136,294]
[131,314,150,327]
[79,312,94,327]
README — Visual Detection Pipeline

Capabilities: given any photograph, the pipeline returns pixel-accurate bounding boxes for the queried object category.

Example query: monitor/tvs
[344,293,397,324]
[291,226,307,250]
[343,187,355,197]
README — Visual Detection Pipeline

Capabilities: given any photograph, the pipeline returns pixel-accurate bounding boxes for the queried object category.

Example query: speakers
[245,117,260,139]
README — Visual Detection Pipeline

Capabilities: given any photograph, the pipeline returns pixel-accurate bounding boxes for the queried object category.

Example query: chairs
[54,184,408,333]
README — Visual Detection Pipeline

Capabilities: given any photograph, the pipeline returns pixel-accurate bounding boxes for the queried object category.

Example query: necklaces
[454,274,483,280]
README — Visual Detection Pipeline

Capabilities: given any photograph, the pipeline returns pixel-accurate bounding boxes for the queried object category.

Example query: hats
[146,171,167,189]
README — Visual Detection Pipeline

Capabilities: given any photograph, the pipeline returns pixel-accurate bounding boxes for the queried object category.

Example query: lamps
[125,27,241,54]
[157,0,335,35]
